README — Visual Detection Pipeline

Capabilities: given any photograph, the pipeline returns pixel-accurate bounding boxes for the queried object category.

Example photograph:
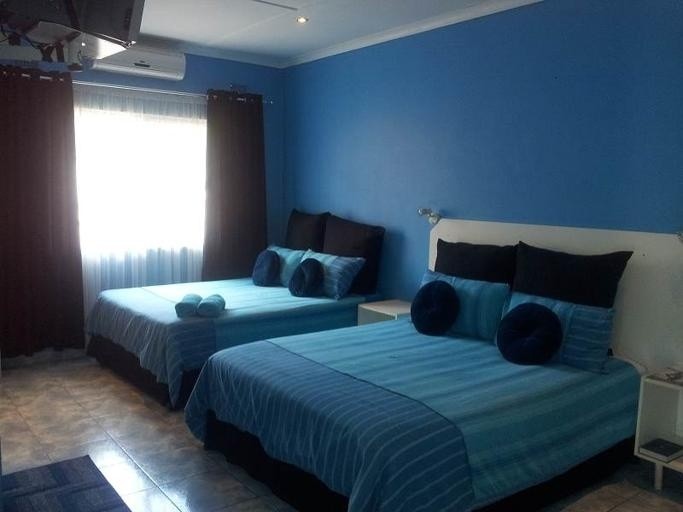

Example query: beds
[86,270,385,406]
[182,219,682,510]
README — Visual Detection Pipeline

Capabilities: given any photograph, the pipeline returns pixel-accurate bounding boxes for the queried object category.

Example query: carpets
[0,453,133,511]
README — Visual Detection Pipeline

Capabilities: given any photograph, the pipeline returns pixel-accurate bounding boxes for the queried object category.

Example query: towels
[196,295,226,319]
[173,294,201,320]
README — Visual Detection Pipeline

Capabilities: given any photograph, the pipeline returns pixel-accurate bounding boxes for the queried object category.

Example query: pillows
[288,258,323,296]
[324,214,384,293]
[496,302,562,365]
[505,292,615,374]
[411,269,509,347]
[250,248,281,287]
[518,242,634,307]
[301,249,367,301]
[255,246,306,288]
[410,280,460,337]
[282,207,332,253]
[435,239,519,289]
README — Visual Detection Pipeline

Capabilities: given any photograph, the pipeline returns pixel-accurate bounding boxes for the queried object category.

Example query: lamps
[416,206,441,226]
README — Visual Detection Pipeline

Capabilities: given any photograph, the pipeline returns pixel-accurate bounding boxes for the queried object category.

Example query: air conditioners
[92,42,189,85]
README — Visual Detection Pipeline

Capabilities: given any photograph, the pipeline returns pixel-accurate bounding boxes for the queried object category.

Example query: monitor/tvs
[20,0,145,66]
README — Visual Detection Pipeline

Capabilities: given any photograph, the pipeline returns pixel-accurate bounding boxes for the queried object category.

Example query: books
[639,437,683,464]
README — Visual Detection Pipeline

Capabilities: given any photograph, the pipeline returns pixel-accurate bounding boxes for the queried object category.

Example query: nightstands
[633,366,682,491]
[356,298,411,328]
